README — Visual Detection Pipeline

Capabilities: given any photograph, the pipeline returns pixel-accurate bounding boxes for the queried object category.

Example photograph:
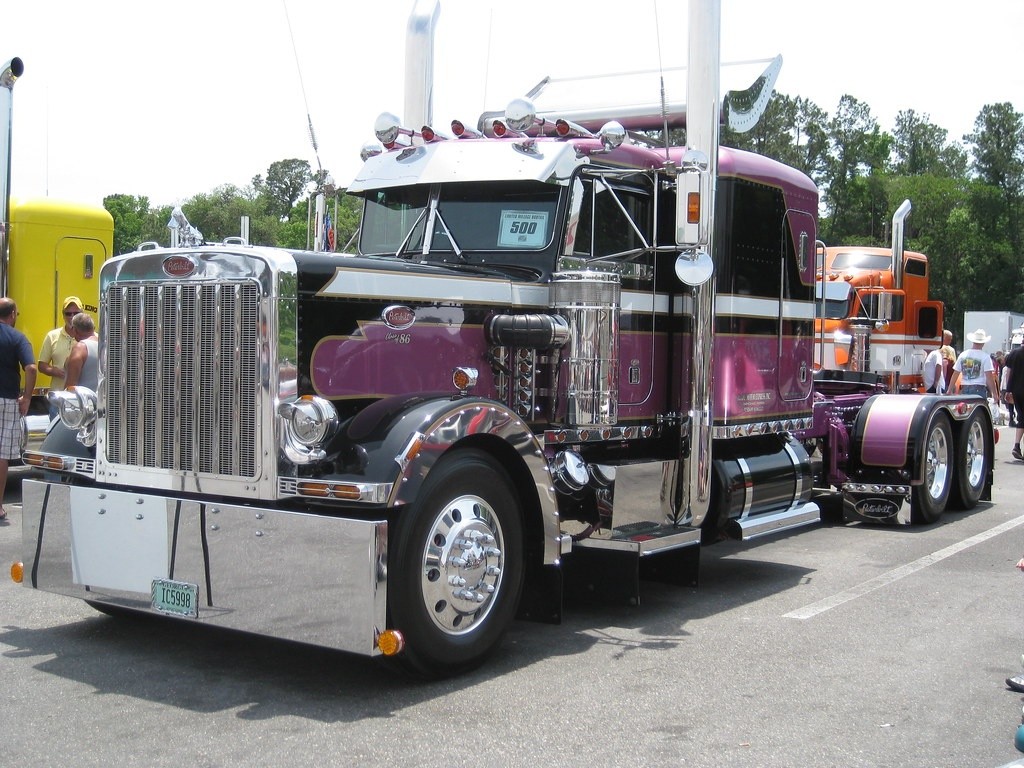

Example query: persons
[38,297,85,422]
[0,297,36,518]
[938,329,957,388]
[921,349,946,395]
[991,334,1024,461]
[948,328,999,404]
[63,314,99,394]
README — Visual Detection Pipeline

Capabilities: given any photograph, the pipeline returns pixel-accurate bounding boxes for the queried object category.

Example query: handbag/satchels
[1004,391,1014,404]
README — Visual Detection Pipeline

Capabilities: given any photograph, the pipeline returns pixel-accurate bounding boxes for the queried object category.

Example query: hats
[62,296,82,310]
[966,329,991,344]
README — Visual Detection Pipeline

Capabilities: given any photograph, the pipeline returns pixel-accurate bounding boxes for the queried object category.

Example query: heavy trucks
[816,197,962,401]
[13,0,1000,681]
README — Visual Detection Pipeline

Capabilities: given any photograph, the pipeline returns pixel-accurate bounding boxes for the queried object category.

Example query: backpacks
[942,357,955,386]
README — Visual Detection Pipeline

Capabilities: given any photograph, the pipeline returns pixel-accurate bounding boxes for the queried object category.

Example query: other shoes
[1005,675,1024,693]
[1011,447,1023,459]
[0,506,7,520]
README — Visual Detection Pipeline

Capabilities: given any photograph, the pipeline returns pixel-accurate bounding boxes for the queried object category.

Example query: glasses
[64,312,80,316]
